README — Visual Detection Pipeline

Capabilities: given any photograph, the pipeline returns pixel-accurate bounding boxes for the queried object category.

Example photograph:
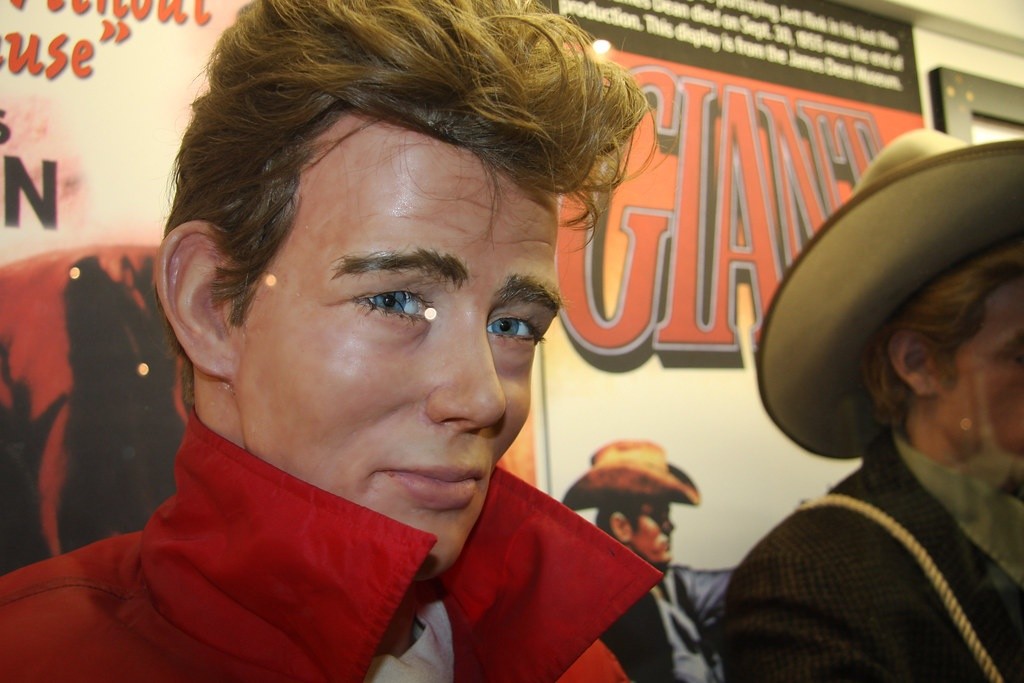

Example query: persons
[0,0,631,683]
[723,128,1024,683]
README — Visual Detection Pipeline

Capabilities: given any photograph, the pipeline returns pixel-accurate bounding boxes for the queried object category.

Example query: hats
[562,440,699,505]
[756,130,1024,459]
